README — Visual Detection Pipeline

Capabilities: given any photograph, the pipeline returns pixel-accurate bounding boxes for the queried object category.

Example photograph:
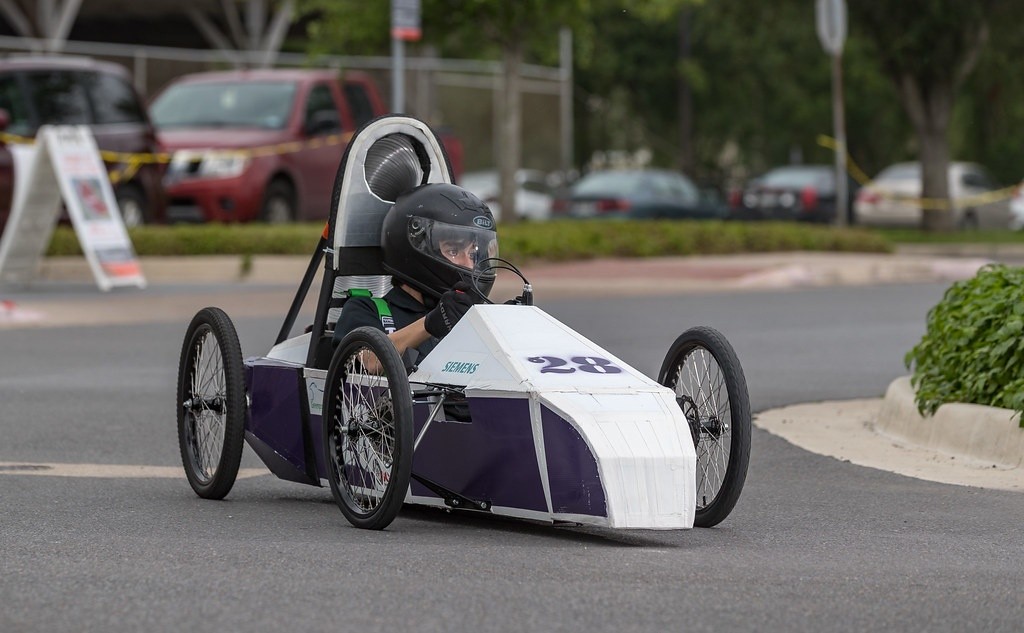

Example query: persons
[329,183,519,377]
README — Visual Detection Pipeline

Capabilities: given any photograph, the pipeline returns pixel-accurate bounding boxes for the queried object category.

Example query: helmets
[382,182,499,307]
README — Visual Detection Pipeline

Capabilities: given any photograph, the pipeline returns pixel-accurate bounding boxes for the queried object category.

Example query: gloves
[425,281,477,338]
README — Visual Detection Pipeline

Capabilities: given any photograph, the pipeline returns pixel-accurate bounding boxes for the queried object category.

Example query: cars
[146,70,466,231]
[852,160,1015,234]
[458,166,551,227]
[729,162,861,225]
[548,166,730,222]
[1,53,169,231]
